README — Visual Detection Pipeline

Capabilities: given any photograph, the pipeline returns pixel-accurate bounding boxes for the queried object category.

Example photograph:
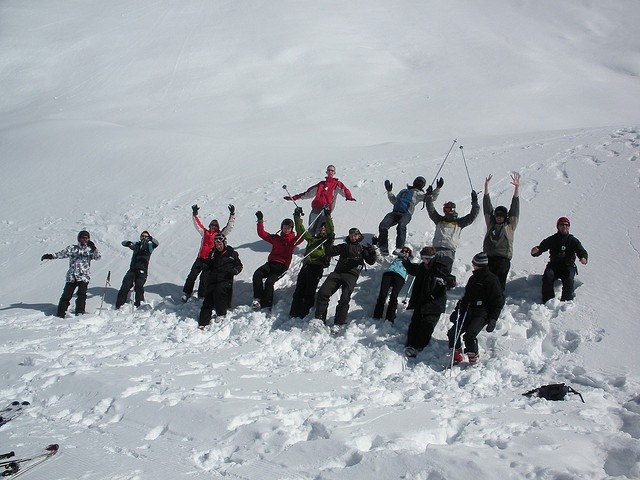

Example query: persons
[371,236,422,324]
[447,252,505,369]
[531,217,589,303]
[41,231,102,319]
[372,175,444,255]
[283,164,357,231]
[251,211,304,312]
[180,203,236,302]
[197,233,244,330]
[289,204,335,322]
[399,248,456,358]
[482,172,520,292]
[424,185,480,274]
[315,227,376,338]
[115,231,159,311]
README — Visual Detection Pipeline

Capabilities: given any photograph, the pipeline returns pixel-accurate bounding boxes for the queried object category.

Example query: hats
[522,384,586,404]
[494,206,507,218]
[472,252,488,266]
[420,247,436,257]
[209,220,220,228]
[77,231,90,241]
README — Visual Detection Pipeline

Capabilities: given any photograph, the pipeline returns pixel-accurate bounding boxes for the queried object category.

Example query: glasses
[443,207,452,213]
[214,236,224,241]
[560,223,569,226]
[400,250,410,253]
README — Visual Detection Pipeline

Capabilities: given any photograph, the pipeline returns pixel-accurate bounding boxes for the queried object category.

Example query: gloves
[426,185,433,194]
[384,180,393,191]
[450,311,457,323]
[325,233,334,245]
[362,242,376,256]
[437,177,444,189]
[191,204,200,215]
[471,190,478,201]
[255,210,263,218]
[87,240,96,251]
[293,207,305,217]
[228,204,235,213]
[321,207,331,217]
[41,253,54,261]
[486,319,496,332]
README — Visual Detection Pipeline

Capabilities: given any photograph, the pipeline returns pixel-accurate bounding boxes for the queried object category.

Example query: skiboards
[0,401,30,428]
[0,444,59,480]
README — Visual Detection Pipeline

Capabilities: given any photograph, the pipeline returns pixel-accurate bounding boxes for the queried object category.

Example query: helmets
[214,234,227,251]
[557,217,570,227]
[349,227,360,235]
[444,202,456,208]
[281,218,294,230]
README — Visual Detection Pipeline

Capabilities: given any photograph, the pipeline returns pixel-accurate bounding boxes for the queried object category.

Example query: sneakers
[463,352,479,370]
[450,348,465,366]
